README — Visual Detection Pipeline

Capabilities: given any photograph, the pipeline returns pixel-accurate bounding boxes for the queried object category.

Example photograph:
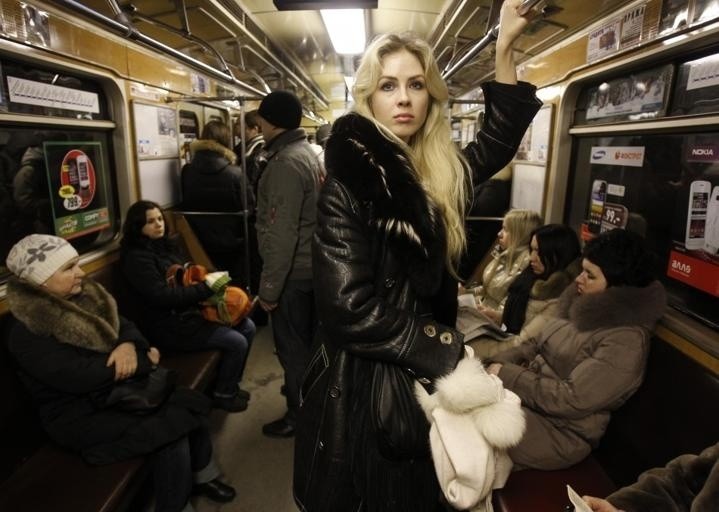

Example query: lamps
[317,9,371,56]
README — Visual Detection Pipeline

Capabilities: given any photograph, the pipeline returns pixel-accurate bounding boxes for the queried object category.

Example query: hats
[259,91,303,130]
[7,234,79,285]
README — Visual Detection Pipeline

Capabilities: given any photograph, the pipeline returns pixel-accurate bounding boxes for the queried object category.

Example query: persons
[116,202,256,412]
[5,233,235,510]
[291,0,543,510]
[179,91,333,438]
[12,130,95,232]
[456,209,665,471]
[567,443,718,511]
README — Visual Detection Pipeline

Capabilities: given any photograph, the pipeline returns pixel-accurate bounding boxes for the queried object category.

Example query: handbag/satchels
[167,262,247,325]
[95,366,174,412]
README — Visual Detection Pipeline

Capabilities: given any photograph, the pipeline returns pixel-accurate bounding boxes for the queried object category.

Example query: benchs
[0,215,260,511]
[493,324,719,511]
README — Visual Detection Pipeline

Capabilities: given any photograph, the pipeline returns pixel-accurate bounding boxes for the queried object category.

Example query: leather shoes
[262,419,293,438]
[196,480,234,502]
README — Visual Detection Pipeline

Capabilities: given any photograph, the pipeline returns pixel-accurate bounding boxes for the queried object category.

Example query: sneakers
[213,389,250,412]
